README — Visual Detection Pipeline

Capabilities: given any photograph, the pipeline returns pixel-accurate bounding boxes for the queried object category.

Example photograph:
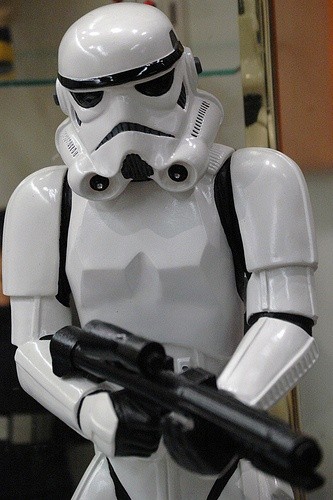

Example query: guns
[51,323,325,490]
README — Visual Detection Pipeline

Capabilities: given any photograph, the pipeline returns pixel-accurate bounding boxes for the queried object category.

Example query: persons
[0,1,321,499]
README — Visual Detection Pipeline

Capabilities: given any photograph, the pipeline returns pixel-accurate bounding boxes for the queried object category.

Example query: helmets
[55,1,225,201]
